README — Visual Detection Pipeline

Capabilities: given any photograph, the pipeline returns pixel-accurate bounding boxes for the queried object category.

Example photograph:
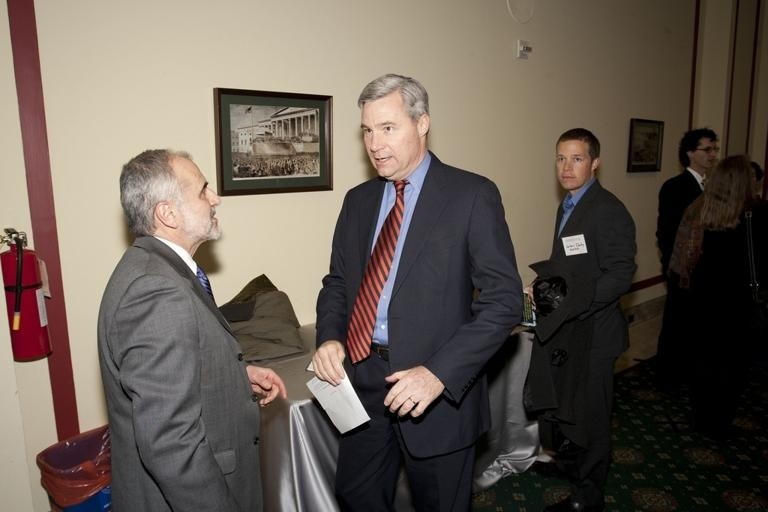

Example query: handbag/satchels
[746,207,767,323]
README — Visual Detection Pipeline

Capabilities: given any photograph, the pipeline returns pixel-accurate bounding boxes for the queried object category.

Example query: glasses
[694,146,722,153]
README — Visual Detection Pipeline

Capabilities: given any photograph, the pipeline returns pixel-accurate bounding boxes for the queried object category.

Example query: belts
[370,343,391,363]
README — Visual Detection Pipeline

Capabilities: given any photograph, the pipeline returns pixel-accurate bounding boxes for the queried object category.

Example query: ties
[195,265,215,299]
[702,179,707,191]
[347,180,410,363]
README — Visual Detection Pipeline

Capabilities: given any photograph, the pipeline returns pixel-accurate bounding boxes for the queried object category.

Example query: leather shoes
[532,461,568,477]
[544,491,606,512]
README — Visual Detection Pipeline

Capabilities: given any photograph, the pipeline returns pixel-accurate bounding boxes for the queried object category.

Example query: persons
[97,150,286,511]
[659,130,722,389]
[316,74,525,510]
[697,155,768,438]
[750,159,764,197]
[540,128,637,511]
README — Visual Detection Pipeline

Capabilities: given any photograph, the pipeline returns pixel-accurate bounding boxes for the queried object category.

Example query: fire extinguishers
[0,228,53,362]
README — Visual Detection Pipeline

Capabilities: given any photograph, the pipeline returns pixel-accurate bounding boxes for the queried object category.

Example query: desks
[230,318,536,512]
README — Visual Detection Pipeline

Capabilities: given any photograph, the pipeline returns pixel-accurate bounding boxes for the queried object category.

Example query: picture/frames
[213,86,335,196]
[626,117,666,172]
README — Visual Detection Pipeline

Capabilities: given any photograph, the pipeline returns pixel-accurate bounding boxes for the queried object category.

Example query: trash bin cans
[36,423,113,512]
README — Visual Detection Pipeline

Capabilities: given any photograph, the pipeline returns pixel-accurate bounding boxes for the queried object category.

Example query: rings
[409,395,416,404]
